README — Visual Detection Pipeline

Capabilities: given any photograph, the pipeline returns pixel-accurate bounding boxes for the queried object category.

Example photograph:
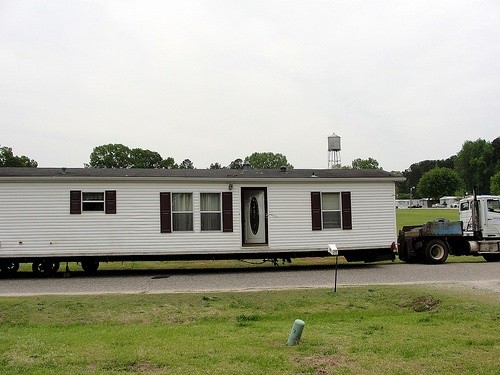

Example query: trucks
[402,194,500,263]
